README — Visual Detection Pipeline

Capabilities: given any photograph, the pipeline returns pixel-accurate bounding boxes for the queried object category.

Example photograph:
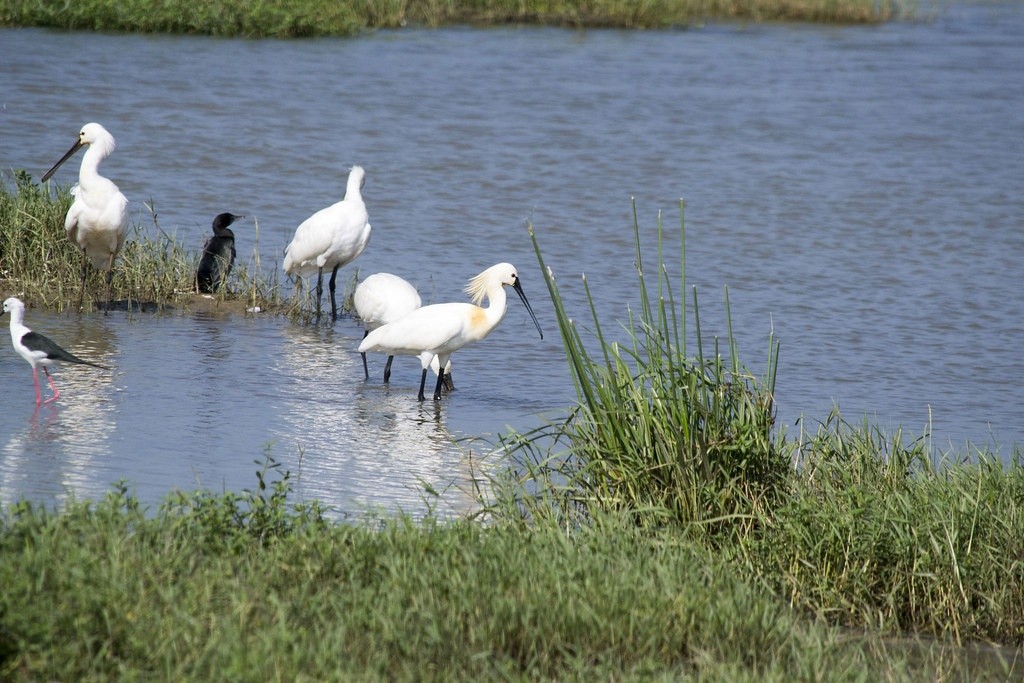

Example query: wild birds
[41,122,130,317]
[282,164,373,322]
[192,212,245,295]
[354,262,544,402]
[0,298,110,407]
